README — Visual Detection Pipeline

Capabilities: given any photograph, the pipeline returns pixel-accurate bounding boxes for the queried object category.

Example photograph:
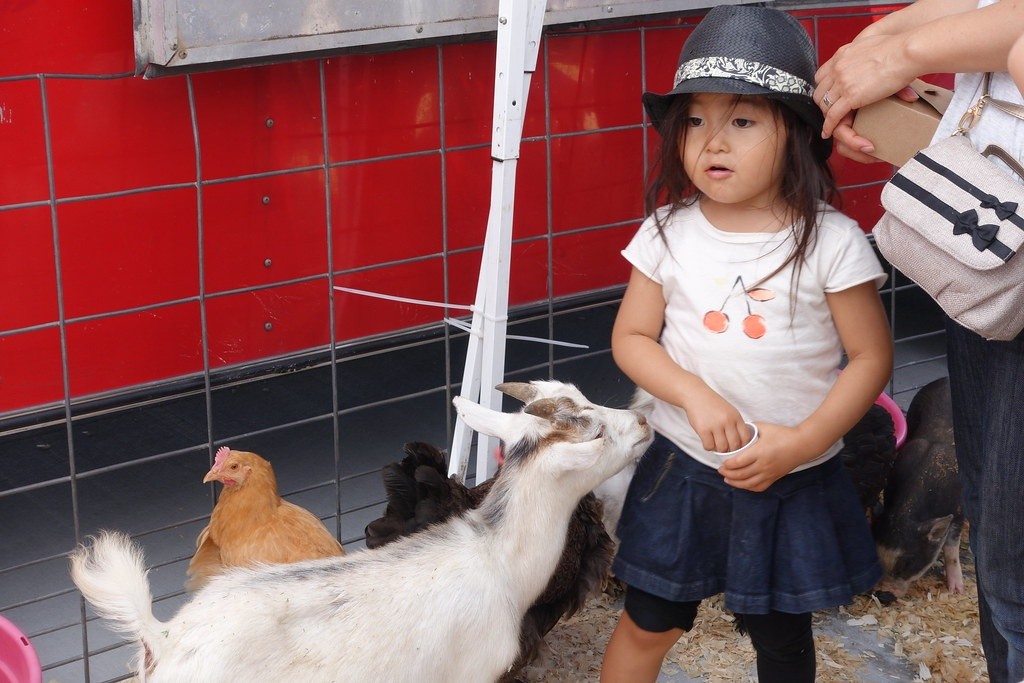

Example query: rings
[823,94,834,109]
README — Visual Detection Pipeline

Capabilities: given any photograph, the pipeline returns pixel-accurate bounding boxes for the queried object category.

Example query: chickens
[363,440,607,683]
[182,441,342,583]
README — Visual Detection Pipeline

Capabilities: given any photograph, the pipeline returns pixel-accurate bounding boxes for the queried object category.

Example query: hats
[641,6,817,135]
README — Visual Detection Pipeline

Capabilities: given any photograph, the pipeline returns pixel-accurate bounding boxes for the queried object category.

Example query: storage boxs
[849,77,955,169]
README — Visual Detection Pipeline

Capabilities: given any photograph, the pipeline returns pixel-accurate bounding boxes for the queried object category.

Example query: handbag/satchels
[871,136,1024,341]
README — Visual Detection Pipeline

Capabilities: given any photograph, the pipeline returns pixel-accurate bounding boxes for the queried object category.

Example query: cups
[712,421,759,464]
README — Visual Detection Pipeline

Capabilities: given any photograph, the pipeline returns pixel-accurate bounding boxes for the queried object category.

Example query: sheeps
[66,377,656,683]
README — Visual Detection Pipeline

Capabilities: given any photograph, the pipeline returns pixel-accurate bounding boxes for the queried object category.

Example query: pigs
[872,374,977,607]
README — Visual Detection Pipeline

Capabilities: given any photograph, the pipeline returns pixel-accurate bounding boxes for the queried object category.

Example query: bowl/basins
[836,368,907,450]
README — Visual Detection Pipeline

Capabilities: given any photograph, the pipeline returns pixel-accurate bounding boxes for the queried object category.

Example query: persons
[597,4,894,683]
[813,0,1024,683]
